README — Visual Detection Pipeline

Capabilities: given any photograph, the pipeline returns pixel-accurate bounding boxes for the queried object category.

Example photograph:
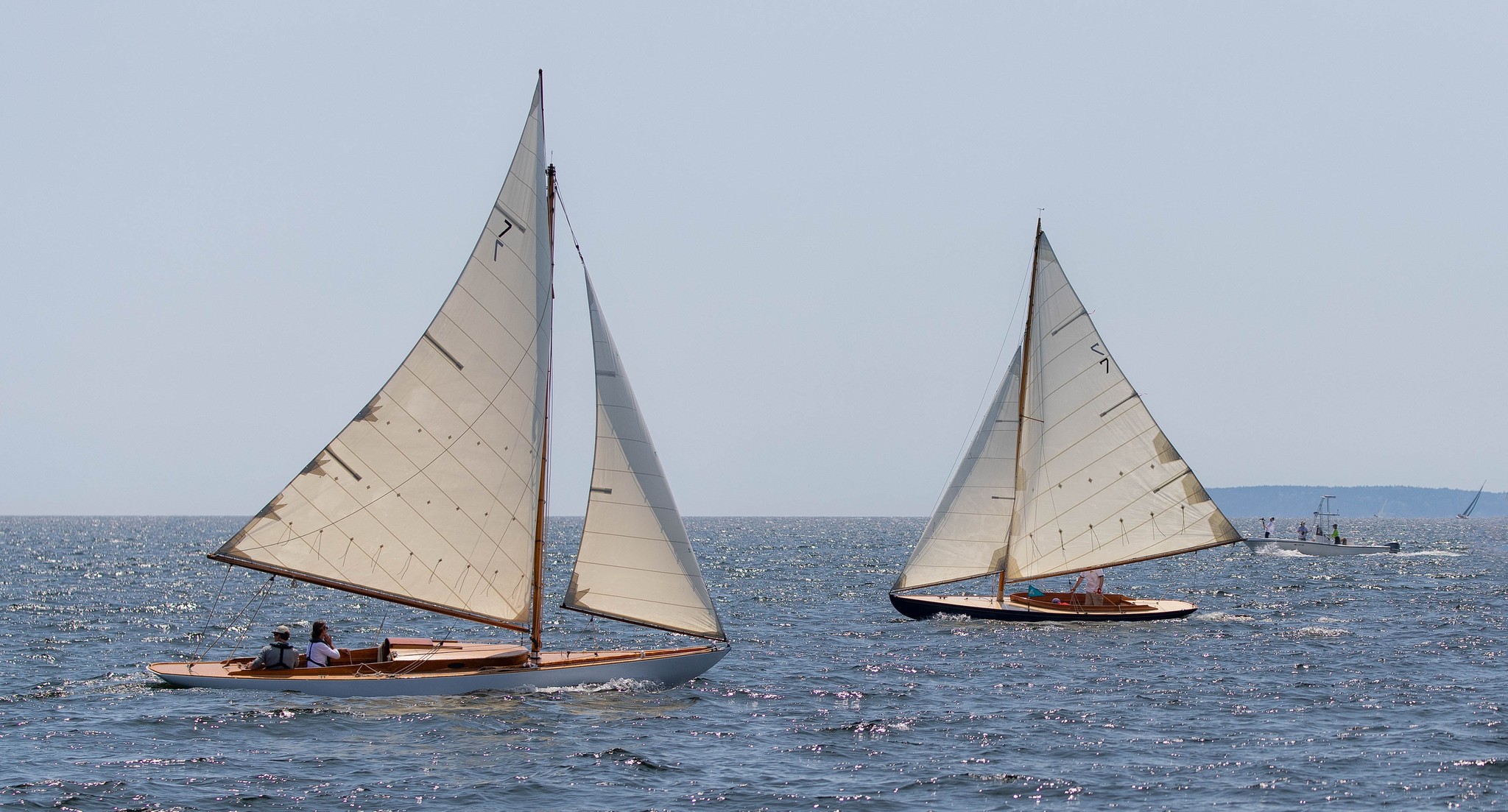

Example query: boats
[1241,494,1399,558]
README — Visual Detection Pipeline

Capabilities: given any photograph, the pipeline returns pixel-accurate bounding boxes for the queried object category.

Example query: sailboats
[886,213,1251,623]
[137,66,735,699]
[1455,479,1488,519]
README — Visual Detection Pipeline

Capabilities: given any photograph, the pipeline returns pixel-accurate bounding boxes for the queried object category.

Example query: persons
[1070,568,1105,606]
[1297,521,1311,541]
[247,625,300,670]
[1264,517,1276,538]
[1327,524,1341,544]
[306,621,350,667]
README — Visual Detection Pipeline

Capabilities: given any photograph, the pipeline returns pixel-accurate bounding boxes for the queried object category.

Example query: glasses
[323,626,328,630]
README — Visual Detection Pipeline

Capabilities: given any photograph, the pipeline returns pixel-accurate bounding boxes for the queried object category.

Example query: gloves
[1070,588,1076,593]
[1096,588,1102,595]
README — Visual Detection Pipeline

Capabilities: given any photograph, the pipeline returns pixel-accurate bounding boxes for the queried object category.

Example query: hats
[271,625,290,634]
[1051,598,1060,603]
